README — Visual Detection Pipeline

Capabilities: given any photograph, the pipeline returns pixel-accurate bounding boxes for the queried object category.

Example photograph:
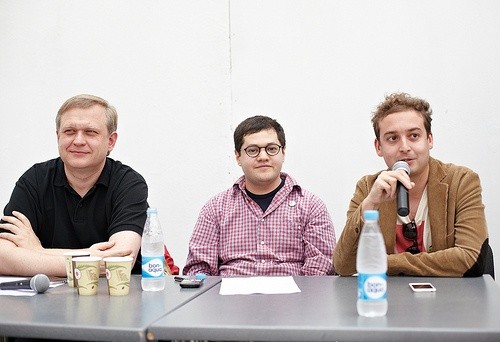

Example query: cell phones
[180,277,203,288]
[408,283,436,292]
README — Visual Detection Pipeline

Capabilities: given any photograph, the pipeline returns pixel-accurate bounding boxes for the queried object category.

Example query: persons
[182,115,337,278]
[0,93,149,276]
[333,92,494,278]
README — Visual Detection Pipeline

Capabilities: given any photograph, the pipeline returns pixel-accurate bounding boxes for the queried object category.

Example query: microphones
[392,161,411,217]
[0,273,50,293]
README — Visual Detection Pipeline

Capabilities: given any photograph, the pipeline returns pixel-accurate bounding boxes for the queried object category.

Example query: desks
[0,275,224,342]
[147,274,500,342]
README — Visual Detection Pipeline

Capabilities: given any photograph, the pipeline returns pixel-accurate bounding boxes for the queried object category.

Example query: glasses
[241,144,284,158]
[402,220,420,254]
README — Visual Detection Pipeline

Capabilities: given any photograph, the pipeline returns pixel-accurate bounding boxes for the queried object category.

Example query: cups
[64,251,91,288]
[103,257,134,295]
[71,257,102,296]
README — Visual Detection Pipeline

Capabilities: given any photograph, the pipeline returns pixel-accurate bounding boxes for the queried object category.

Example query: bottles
[141,207,165,291]
[356,210,388,318]
